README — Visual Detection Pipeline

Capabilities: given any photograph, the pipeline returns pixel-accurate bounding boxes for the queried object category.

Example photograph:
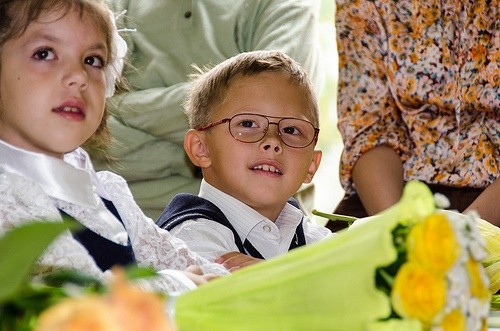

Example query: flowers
[174,178,492,331]
[0,217,178,331]
[312,207,500,311]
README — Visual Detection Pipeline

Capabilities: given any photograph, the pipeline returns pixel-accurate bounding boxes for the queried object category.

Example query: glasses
[197,112,320,149]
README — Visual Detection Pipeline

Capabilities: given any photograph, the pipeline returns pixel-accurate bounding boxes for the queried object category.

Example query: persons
[153,49,335,277]
[324,0,500,238]
[80,0,326,229]
[1,0,236,310]
